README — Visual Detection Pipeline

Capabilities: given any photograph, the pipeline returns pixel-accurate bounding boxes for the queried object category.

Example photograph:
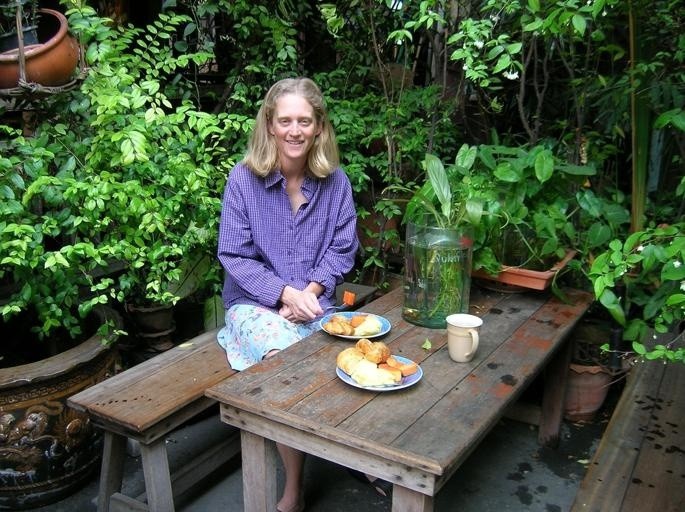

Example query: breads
[325,312,384,337]
[336,338,416,390]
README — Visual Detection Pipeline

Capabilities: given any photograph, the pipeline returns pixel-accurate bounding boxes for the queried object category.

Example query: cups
[446,313,483,363]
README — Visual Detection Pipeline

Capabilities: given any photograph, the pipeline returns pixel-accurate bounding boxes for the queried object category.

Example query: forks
[322,302,349,313]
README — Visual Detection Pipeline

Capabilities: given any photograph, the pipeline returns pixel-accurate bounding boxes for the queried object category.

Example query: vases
[356,211,396,252]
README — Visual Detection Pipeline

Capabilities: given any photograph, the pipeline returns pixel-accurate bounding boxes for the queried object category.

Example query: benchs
[69,282,379,512]
[569,330,685,512]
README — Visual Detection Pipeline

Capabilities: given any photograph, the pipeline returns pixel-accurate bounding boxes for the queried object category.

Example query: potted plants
[0,123,129,512]
[399,145,519,326]
[0,0,79,88]
[20,0,261,367]
[557,191,630,421]
[448,140,628,305]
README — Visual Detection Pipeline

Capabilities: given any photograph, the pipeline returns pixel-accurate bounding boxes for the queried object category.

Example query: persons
[216,76,391,512]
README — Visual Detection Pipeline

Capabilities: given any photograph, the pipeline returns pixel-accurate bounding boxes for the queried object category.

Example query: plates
[319,311,391,339]
[336,354,423,391]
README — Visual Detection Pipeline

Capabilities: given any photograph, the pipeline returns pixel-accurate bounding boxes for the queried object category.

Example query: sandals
[350,465,392,499]
